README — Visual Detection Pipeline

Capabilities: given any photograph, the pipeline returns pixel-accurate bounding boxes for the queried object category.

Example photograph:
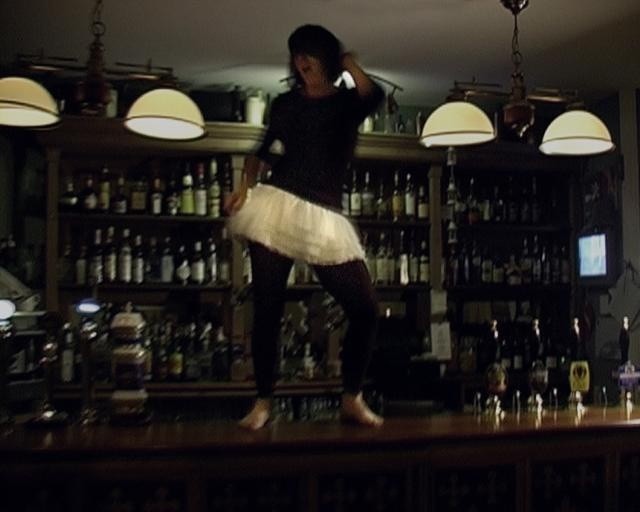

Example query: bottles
[448,167,562,228]
[52,316,106,390]
[483,318,507,398]
[243,237,433,289]
[563,318,592,401]
[59,148,233,218]
[443,237,570,292]
[143,318,229,383]
[71,230,232,289]
[245,162,430,227]
[524,318,548,395]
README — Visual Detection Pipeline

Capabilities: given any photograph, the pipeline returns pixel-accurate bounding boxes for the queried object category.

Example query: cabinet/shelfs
[433,157,583,375]
[44,146,242,386]
[242,154,435,379]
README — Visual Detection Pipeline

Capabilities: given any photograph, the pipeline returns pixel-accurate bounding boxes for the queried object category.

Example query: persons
[220,22,390,431]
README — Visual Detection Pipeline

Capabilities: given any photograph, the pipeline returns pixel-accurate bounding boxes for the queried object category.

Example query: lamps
[418,0,616,156]
[0,0,207,141]
